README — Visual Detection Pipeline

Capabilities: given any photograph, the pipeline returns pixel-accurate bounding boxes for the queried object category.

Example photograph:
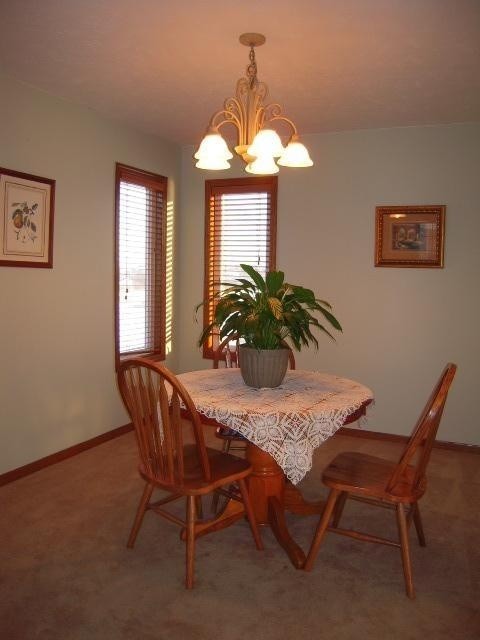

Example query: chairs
[304,360,458,598]
[206,334,302,516]
[109,360,268,593]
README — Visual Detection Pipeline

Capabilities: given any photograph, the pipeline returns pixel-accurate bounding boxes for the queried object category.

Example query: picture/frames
[0,166,56,268]
[374,205,446,269]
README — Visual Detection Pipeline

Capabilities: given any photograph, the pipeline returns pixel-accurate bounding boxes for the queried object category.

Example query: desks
[160,364,378,570]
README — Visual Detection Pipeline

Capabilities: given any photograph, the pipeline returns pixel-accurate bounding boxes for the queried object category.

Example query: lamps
[193,35,315,175]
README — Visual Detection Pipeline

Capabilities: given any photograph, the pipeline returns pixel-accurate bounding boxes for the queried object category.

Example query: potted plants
[191,263,344,388]
